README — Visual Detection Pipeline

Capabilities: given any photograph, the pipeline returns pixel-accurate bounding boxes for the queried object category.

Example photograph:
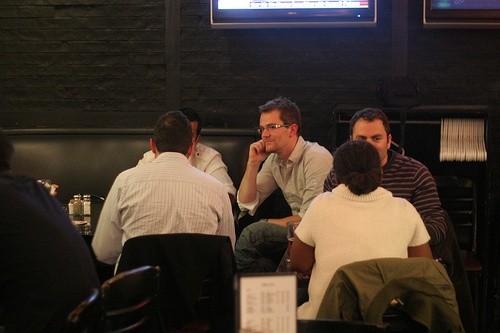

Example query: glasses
[255,124,290,135]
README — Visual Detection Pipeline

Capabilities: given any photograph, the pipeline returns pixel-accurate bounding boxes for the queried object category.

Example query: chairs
[316,257,465,333]
[66,233,238,333]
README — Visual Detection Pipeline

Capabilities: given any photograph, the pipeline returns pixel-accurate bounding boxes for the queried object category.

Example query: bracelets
[260,219,266,222]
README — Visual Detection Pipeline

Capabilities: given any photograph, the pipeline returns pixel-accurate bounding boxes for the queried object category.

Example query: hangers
[440,119,487,162]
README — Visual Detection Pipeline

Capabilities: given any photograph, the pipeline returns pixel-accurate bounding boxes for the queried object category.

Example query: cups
[287,223,299,256]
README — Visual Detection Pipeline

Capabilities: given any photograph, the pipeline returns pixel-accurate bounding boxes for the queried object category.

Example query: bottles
[83,195,91,216]
[68,200,74,215]
[73,195,83,216]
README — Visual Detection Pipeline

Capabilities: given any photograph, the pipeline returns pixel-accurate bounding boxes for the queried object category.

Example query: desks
[65,203,104,235]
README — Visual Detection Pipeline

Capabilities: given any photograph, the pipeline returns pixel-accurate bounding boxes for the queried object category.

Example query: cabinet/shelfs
[334,104,490,252]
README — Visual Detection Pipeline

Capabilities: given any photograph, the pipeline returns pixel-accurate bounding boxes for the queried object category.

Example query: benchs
[0,128,260,201]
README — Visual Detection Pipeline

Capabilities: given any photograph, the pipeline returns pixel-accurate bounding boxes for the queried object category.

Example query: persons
[290,139,434,320]
[0,134,101,333]
[91,111,238,275]
[322,108,451,248]
[234,97,334,274]
[136,106,238,209]
